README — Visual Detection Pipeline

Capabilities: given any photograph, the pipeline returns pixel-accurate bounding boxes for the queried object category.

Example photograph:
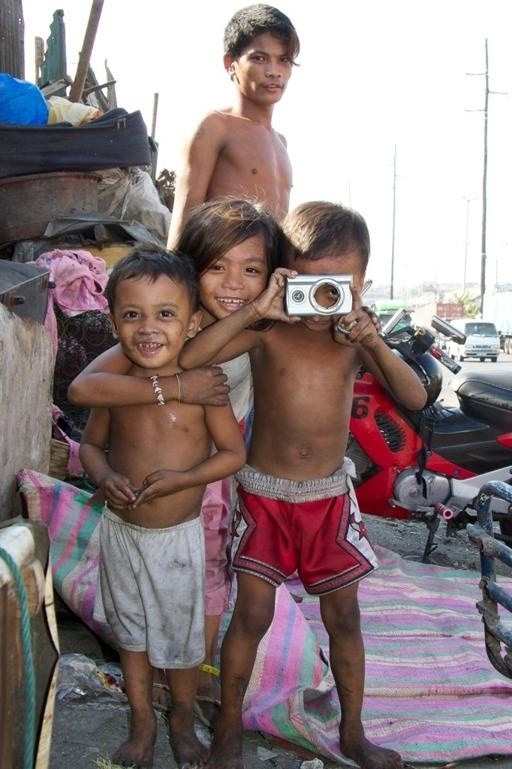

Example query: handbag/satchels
[0,108,158,179]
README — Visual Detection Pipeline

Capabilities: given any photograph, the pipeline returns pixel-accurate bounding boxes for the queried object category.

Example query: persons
[77,239,247,767]
[67,188,385,734]
[173,197,430,767]
[162,2,303,262]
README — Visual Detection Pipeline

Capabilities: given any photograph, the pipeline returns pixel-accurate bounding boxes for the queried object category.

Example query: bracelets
[174,372,184,404]
[150,373,165,408]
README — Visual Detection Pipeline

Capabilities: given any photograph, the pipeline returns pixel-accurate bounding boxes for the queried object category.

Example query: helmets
[390,352,443,409]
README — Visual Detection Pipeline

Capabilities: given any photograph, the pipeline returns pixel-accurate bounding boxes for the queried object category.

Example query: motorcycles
[342,300,512,566]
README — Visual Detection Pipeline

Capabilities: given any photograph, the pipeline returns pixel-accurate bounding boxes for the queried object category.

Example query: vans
[443,317,502,362]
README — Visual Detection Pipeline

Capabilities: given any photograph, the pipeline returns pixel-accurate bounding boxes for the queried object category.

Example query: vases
[1,170,102,241]
